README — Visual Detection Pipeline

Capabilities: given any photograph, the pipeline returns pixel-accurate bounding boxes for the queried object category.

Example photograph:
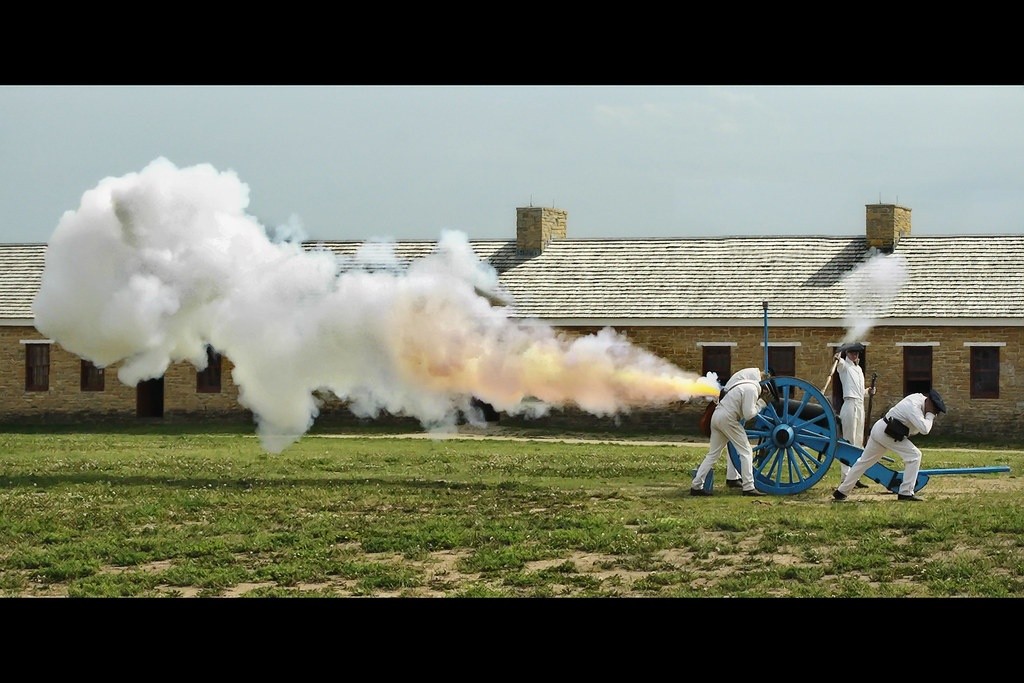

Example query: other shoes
[898,494,923,501]
[690,488,713,496]
[856,481,868,488]
[742,488,766,496]
[726,479,742,487]
[833,490,847,500]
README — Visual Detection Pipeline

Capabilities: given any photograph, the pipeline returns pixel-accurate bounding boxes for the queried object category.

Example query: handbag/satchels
[701,400,717,437]
[884,417,909,442]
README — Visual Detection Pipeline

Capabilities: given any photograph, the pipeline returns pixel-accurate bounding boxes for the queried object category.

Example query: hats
[929,389,946,413]
[847,343,864,352]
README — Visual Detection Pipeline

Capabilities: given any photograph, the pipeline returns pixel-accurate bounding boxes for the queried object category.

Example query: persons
[832,390,946,501]
[834,342,875,489]
[721,367,775,487]
[690,378,779,496]
